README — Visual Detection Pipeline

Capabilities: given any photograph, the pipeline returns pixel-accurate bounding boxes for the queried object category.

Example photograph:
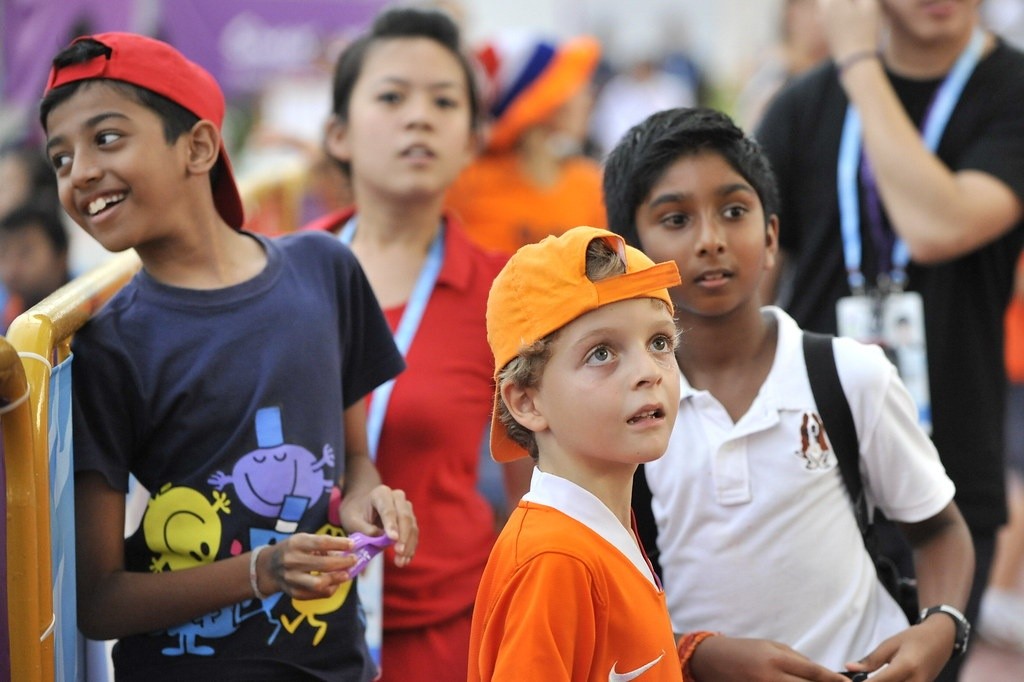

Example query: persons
[601,106,975,682]
[37,31,419,682]
[297,8,534,682]
[1,0,1024,654]
[467,225,682,682]
[754,1,1023,682]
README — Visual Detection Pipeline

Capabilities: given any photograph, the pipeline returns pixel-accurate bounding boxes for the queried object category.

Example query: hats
[486,226,682,464]
[44,32,244,231]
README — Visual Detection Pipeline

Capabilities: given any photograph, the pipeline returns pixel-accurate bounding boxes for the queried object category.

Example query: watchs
[919,603,971,661]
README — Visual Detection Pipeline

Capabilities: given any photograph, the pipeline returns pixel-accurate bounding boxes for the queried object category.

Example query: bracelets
[676,629,715,668]
[249,544,272,600]
